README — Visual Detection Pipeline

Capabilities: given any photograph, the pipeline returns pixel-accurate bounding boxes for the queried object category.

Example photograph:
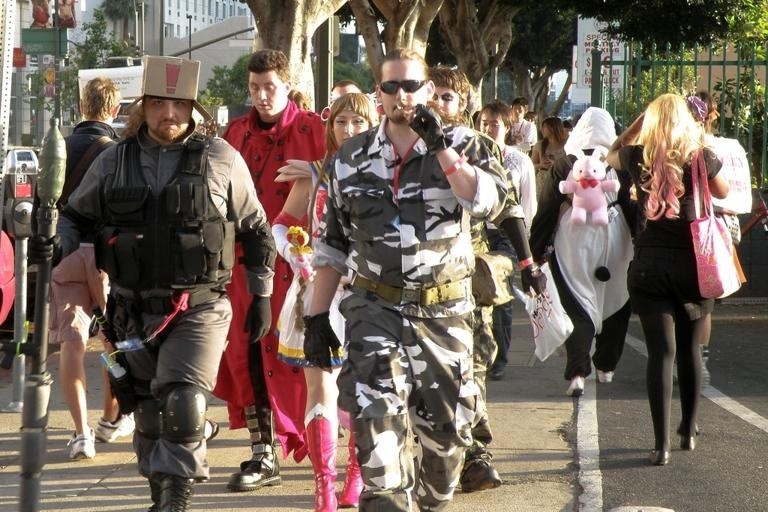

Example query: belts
[351,273,470,305]
[112,287,226,314]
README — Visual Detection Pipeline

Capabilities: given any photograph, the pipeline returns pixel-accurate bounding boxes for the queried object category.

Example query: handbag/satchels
[687,150,742,299]
[471,251,516,307]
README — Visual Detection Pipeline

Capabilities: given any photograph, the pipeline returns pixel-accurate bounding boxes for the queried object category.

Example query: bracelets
[518,256,534,270]
[443,149,468,177]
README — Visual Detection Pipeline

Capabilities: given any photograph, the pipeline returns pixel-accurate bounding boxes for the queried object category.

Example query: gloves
[521,265,548,298]
[304,313,339,370]
[409,103,453,156]
[30,234,61,266]
[244,296,272,345]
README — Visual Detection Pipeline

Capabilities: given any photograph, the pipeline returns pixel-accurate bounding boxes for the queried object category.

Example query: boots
[305,403,339,511]
[159,478,192,512]
[146,479,161,512]
[229,404,280,489]
[339,415,365,508]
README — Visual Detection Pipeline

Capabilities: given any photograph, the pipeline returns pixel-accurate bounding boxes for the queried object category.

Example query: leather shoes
[487,365,505,381]
[460,462,500,491]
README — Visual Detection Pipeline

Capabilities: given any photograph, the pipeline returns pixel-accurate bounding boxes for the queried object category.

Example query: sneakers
[568,376,586,396]
[652,447,671,466]
[95,417,135,442]
[69,428,96,458]
[680,432,695,449]
[598,370,612,383]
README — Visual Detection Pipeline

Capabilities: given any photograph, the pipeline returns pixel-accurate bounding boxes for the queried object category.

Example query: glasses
[380,79,427,93]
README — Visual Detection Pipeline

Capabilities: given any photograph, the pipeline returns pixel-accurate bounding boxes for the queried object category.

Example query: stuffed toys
[559,143,622,226]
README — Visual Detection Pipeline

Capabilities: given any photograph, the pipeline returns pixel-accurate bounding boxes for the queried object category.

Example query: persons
[32,78,136,461]
[507,97,537,154]
[408,68,548,492]
[269,93,375,512]
[606,92,731,465]
[330,80,364,106]
[27,56,276,512]
[672,91,752,388]
[530,107,634,397]
[479,102,538,382]
[222,48,327,491]
[530,117,568,203]
[301,48,509,512]
